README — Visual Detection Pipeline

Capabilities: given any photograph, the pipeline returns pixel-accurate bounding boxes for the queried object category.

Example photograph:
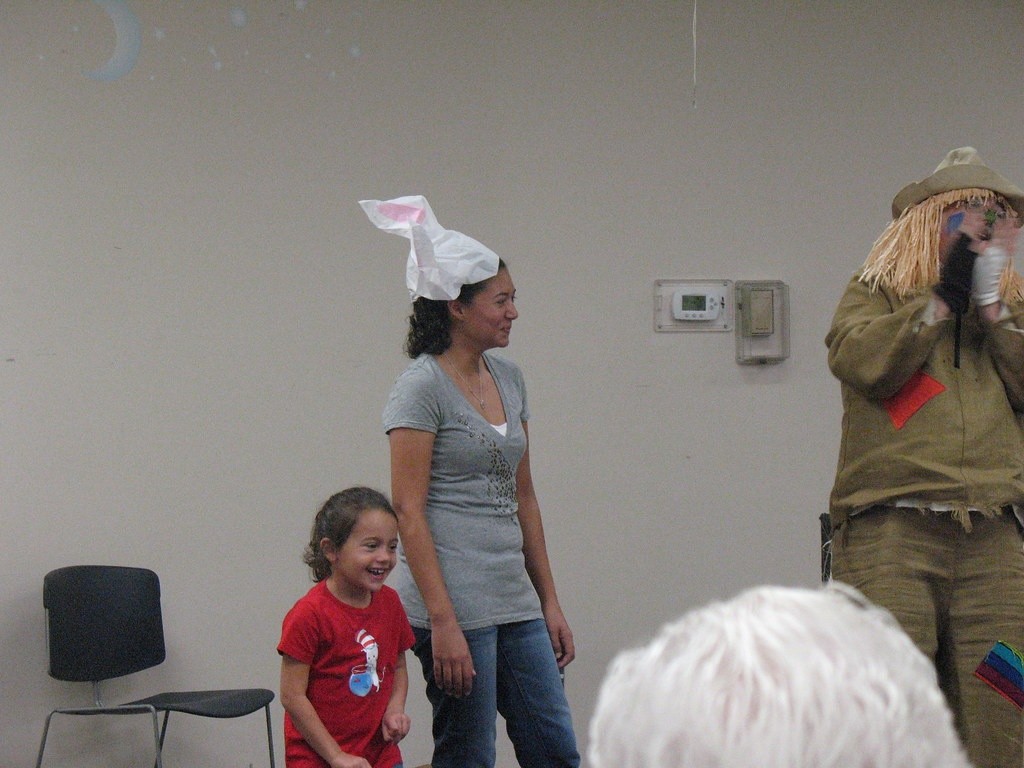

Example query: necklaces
[443,352,485,410]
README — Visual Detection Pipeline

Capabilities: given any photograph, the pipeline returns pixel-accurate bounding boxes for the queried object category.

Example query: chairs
[36,566,275,768]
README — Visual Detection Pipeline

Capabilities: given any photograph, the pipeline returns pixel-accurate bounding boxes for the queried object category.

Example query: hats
[894,147,1024,229]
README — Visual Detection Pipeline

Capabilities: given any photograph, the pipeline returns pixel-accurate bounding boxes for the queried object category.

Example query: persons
[359,194,582,768]
[277,487,417,768]
[825,147,1024,768]
[589,584,965,768]
